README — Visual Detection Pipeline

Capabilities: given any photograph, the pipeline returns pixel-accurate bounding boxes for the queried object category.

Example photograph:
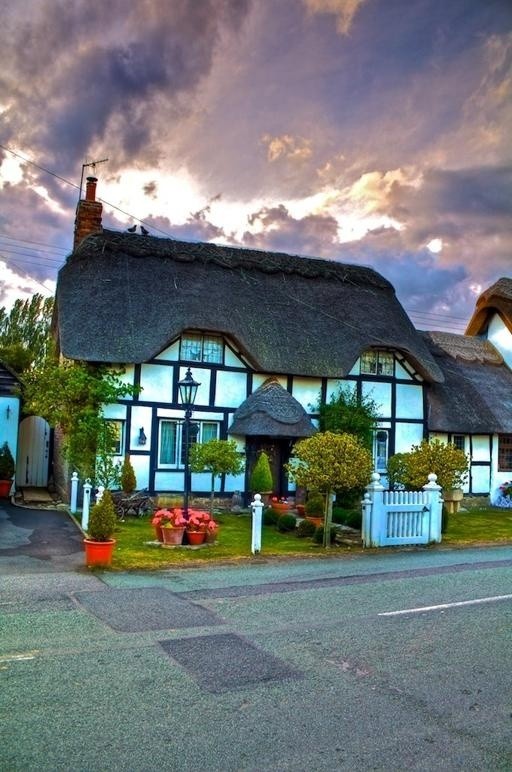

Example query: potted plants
[82,489,119,569]
[304,494,325,532]
[0,440,16,498]
[250,449,274,506]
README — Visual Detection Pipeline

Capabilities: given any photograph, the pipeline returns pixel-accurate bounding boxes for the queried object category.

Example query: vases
[272,503,306,516]
[152,522,217,547]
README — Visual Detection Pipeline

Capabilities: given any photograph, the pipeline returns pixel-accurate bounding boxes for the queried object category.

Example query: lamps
[138,426,147,445]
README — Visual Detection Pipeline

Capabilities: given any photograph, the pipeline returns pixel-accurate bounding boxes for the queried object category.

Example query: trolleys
[109,487,156,522]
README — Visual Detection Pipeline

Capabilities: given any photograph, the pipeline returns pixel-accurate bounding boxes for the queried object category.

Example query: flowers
[149,505,219,537]
[271,495,288,504]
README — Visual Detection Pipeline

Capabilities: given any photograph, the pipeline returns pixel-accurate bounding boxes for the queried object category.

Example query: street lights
[175,365,204,526]
